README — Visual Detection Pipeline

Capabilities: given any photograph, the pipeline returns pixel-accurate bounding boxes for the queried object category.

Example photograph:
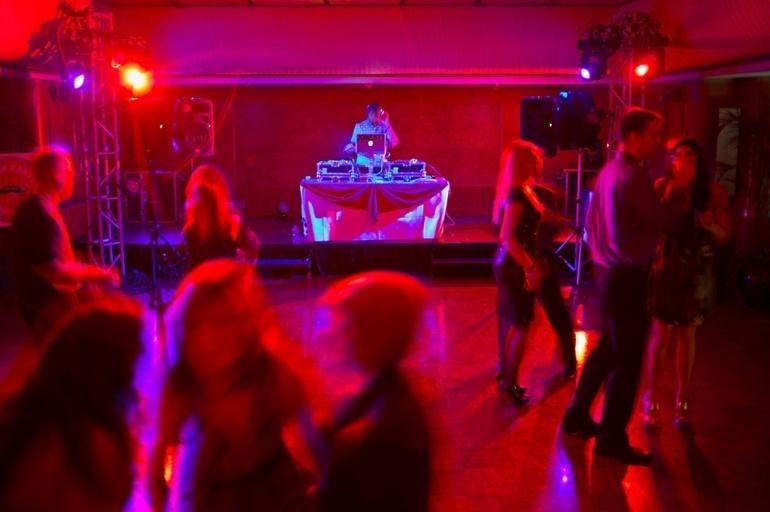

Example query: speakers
[560,92,597,149]
[520,96,561,157]
[174,97,215,159]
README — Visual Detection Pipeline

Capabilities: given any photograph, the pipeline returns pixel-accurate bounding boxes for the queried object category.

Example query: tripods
[553,150,592,288]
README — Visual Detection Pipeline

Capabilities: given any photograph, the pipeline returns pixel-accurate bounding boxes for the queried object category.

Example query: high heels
[673,395,691,428]
[642,392,659,427]
[496,375,528,405]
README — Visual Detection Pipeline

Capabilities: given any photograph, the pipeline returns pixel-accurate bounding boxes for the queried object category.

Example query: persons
[145,256,310,512]
[0,295,147,512]
[641,139,732,430]
[183,166,261,269]
[0,143,121,323]
[344,102,399,166]
[562,105,664,462]
[302,270,427,512]
[493,139,576,406]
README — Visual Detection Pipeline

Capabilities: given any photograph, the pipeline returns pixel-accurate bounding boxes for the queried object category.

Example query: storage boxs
[387,161,425,176]
[317,160,353,175]
[564,170,597,214]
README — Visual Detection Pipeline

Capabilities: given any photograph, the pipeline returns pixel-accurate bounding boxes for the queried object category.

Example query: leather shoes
[593,441,651,464]
[561,414,601,432]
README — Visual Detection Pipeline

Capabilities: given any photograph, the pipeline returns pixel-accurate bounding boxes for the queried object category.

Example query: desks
[304,180,449,237]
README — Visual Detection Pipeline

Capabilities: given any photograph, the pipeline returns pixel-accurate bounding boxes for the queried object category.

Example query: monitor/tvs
[357,134,386,155]
